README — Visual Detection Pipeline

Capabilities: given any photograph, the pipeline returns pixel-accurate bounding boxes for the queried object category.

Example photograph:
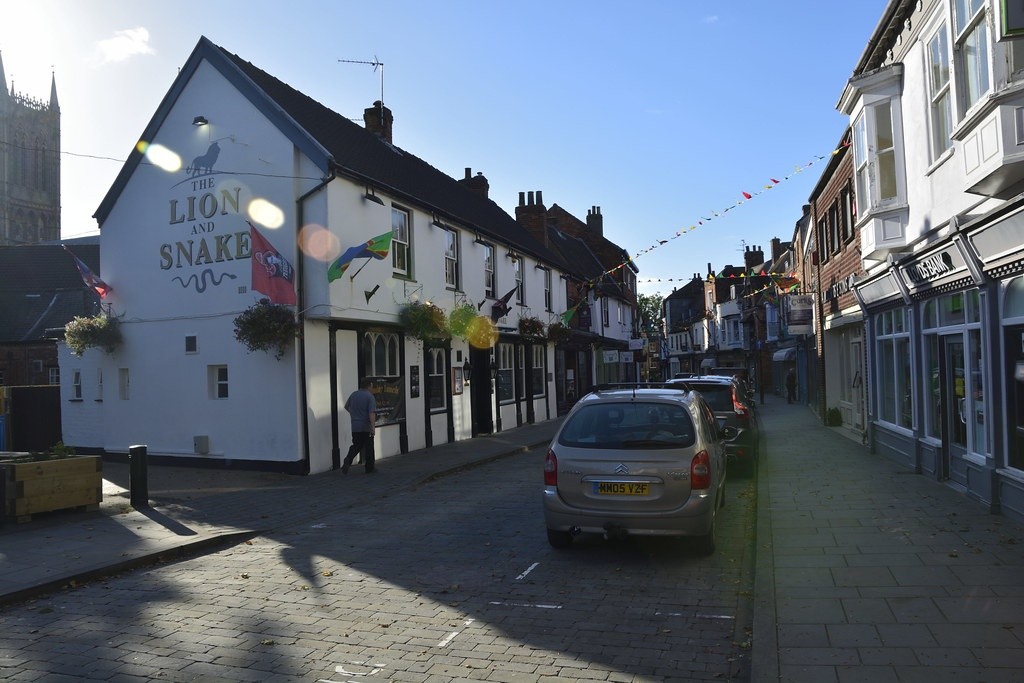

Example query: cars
[536,384,728,558]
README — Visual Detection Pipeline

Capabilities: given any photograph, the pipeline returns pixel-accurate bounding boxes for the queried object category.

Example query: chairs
[607,406,625,434]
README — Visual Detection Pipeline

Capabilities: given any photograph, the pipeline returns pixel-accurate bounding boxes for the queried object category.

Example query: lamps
[463,356,472,380]
[490,357,498,379]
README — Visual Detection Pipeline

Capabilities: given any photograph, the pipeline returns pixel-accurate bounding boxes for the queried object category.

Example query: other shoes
[365,468,376,474]
[341,467,348,477]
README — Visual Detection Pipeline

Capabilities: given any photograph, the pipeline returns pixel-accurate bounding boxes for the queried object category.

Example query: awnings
[771,348,798,362]
[700,357,717,369]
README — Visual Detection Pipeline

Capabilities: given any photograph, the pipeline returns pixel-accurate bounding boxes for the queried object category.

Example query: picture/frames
[452,366,463,395]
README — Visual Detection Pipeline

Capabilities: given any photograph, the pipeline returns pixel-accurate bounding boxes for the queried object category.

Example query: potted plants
[0,441,104,524]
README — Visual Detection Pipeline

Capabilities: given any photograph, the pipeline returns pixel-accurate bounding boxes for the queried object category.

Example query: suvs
[664,370,754,479]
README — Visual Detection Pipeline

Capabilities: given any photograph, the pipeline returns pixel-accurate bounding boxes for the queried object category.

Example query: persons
[785,368,799,404]
[340,379,378,475]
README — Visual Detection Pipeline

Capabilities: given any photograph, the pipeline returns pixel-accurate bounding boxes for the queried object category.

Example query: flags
[326,231,394,283]
[63,245,114,300]
[559,297,587,325]
[769,275,800,295]
[250,223,299,306]
[491,285,519,324]
